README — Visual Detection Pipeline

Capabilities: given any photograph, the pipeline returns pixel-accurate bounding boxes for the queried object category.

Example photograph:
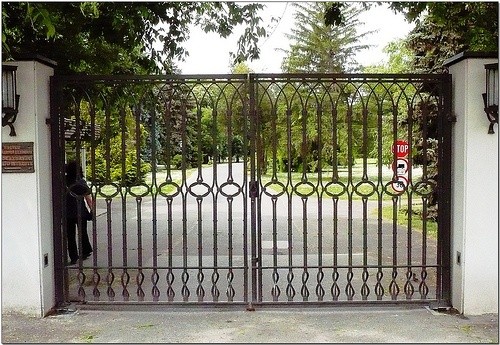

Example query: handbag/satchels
[84,195,92,221]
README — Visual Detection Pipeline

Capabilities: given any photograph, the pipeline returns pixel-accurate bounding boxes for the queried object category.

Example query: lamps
[2,64,22,137]
[481,62,498,133]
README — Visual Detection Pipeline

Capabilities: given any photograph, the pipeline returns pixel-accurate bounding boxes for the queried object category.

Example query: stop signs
[391,140,410,158]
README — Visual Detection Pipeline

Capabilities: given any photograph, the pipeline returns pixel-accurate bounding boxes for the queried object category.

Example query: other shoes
[71,259,77,264]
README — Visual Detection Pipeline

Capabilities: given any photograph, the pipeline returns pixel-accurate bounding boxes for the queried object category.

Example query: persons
[64,160,94,265]
